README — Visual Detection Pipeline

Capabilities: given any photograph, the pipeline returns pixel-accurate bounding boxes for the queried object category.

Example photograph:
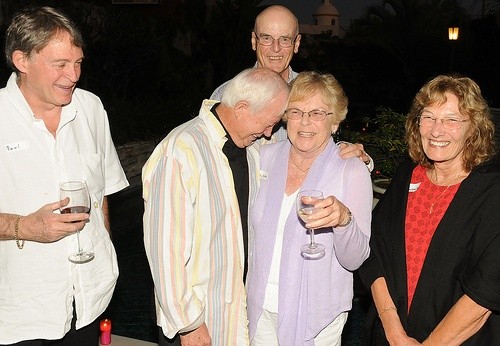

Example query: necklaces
[289,153,313,172]
[429,169,464,213]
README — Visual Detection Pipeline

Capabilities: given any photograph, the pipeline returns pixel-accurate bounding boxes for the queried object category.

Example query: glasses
[251,29,297,47]
[415,114,472,126]
[284,108,334,120]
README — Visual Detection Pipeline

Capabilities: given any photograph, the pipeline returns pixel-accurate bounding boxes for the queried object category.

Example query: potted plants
[333,104,410,198]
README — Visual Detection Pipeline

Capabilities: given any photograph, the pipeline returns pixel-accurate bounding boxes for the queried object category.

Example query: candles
[100,319,112,345]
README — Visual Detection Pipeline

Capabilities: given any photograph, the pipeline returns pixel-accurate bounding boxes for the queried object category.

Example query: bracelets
[378,306,397,317]
[339,212,352,226]
[15,216,25,249]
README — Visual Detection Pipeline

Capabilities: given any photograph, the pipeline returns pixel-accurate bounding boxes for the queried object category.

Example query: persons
[142,68,375,346]
[359,76,500,346]
[210,5,302,146]
[247,72,373,346]
[1,8,129,346]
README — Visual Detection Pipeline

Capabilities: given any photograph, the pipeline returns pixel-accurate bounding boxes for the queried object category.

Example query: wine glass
[58,180,95,263]
[296,190,326,254]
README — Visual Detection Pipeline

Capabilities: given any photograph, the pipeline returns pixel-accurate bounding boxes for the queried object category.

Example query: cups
[100,319,111,344]
